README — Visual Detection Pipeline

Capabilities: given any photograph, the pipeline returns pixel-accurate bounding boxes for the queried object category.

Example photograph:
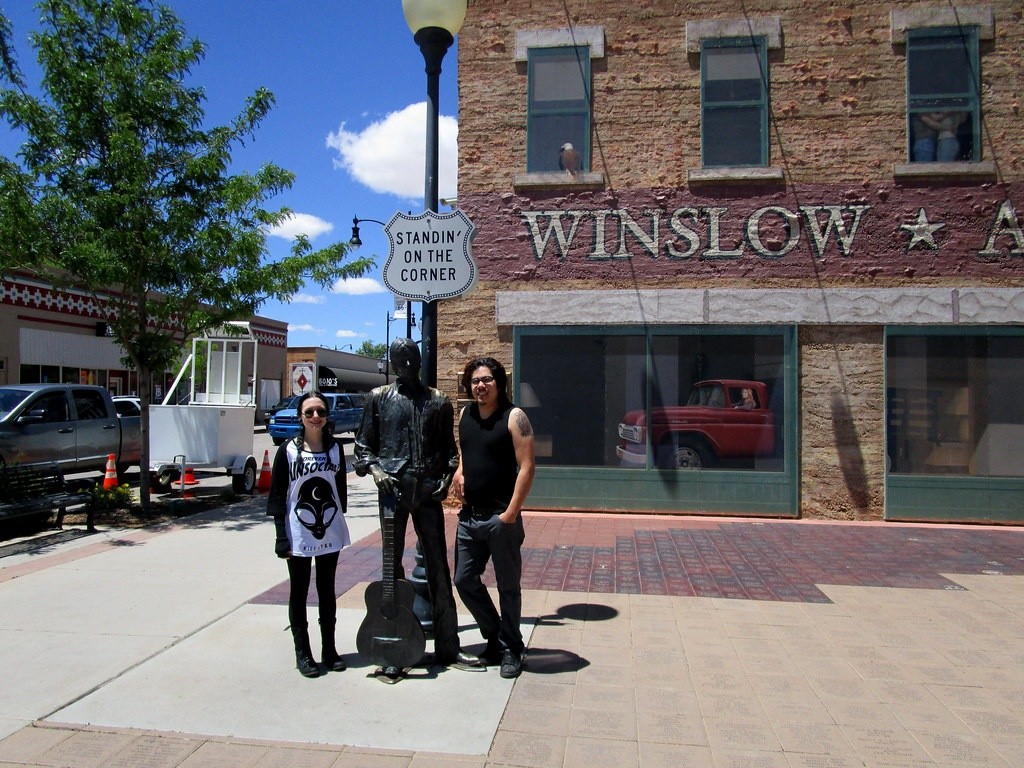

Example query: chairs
[42,396,68,423]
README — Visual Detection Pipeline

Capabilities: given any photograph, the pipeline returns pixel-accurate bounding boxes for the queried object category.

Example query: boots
[290,622,320,676]
[319,616,345,670]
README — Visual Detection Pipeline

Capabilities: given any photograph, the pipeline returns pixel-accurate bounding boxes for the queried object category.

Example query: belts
[462,505,495,517]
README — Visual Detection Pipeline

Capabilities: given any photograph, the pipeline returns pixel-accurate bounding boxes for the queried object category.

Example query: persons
[732,389,757,411]
[267,391,352,678]
[353,339,487,679]
[456,358,535,679]
[910,71,967,162]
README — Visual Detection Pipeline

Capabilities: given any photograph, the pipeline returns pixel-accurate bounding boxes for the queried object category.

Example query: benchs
[0,461,98,534]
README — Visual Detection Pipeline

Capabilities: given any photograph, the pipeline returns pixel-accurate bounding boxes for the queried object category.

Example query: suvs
[267,392,368,446]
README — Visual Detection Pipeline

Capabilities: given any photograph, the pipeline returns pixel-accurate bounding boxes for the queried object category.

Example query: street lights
[349,212,423,344]
[377,311,417,385]
[403,1,469,641]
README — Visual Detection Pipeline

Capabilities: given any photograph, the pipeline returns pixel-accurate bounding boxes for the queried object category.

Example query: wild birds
[559,143,583,178]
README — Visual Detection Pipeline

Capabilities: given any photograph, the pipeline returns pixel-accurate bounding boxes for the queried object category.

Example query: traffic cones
[257,449,272,495]
[102,453,120,492]
[174,467,201,485]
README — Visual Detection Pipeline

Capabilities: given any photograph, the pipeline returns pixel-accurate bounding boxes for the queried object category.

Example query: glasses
[301,410,327,418]
[469,376,494,385]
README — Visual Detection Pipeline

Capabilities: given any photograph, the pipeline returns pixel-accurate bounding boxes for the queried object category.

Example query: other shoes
[500,649,522,677]
[479,635,502,666]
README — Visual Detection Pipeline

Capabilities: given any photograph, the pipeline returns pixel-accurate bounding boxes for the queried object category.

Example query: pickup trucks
[616,379,781,470]
[0,380,142,499]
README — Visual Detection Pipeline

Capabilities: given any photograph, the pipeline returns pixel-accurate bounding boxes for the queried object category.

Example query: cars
[264,394,298,432]
[110,394,141,417]
